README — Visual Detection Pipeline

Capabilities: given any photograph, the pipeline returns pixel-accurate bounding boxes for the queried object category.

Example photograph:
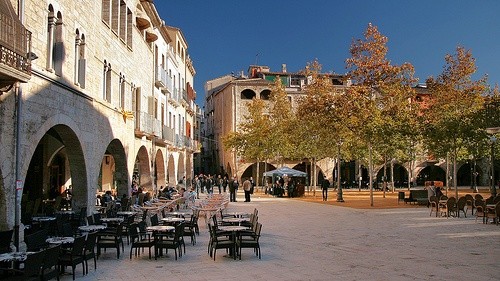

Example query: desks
[161,217,184,226]
[117,211,135,215]
[169,212,191,217]
[223,218,250,226]
[33,216,56,221]
[487,204,497,207]
[219,226,247,257]
[0,252,36,269]
[224,211,254,218]
[140,206,157,210]
[440,200,448,203]
[100,218,123,222]
[57,210,79,214]
[46,237,74,244]
[146,226,175,256]
[78,225,105,231]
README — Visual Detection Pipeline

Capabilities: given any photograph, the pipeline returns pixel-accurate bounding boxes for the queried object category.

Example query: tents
[263,166,307,197]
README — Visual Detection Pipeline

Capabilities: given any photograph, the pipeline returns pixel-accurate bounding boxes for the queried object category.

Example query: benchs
[404,190,442,205]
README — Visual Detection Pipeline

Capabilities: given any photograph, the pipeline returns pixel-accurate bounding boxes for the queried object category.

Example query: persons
[436,187,446,208]
[249,177,253,194]
[427,181,436,208]
[321,176,330,201]
[216,175,222,193]
[275,176,297,198]
[222,176,227,192]
[228,177,239,202]
[101,191,112,206]
[65,185,72,208]
[133,186,137,193]
[192,174,214,199]
[242,177,252,202]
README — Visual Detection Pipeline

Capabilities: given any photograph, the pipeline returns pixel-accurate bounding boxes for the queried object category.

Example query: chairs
[398,192,405,203]
[0,189,262,281]
[429,194,500,226]
